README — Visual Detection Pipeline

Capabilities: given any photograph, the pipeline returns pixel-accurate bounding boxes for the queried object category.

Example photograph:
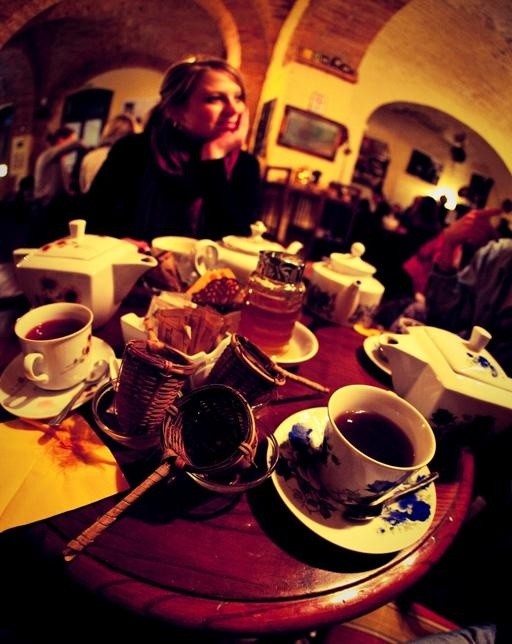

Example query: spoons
[342,472,439,520]
[47,360,109,428]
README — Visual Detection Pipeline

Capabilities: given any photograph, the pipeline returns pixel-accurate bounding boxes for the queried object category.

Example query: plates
[362,333,399,378]
[0,336,119,421]
[218,308,319,369]
[266,405,440,558]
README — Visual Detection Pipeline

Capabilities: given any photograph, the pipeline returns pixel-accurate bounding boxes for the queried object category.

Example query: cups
[13,302,95,392]
[317,384,437,506]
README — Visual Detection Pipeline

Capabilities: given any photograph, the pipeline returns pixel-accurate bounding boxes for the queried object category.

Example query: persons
[29,53,270,239]
[305,170,511,374]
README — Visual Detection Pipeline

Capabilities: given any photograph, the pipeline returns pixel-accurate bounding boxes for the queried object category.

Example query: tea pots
[379,324,512,444]
[13,219,159,331]
[193,221,305,286]
[309,239,385,329]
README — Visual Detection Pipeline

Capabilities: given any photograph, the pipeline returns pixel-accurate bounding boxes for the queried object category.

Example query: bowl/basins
[149,234,202,291]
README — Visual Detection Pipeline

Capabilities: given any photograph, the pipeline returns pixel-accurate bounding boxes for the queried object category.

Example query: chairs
[245,163,372,248]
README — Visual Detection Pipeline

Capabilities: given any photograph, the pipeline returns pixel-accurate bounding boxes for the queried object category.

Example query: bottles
[237,250,307,351]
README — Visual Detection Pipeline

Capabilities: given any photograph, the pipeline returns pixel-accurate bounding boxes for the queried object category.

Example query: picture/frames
[277,101,348,164]
